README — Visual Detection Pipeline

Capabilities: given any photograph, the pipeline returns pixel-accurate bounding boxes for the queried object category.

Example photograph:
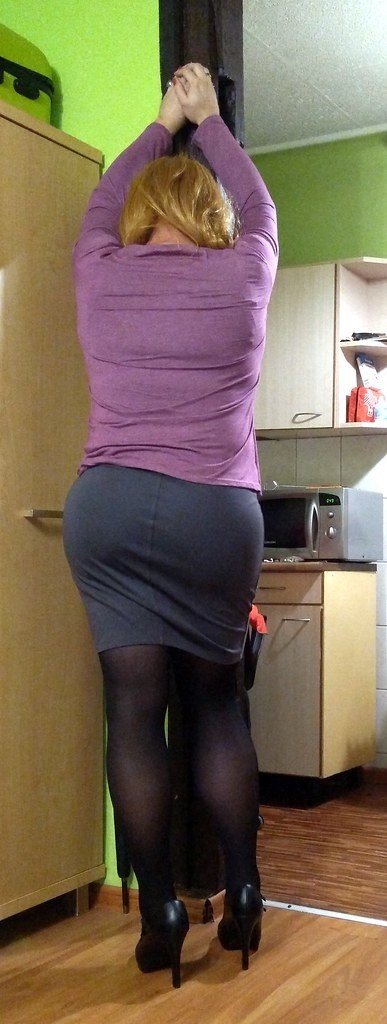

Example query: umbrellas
[114,810,131,914]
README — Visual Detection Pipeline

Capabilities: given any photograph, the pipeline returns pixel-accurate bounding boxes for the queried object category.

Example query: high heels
[135,900,190,988]
[218,882,266,970]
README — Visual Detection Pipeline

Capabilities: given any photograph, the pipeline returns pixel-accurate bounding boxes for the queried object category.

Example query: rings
[169,81,172,86]
[205,72,209,75]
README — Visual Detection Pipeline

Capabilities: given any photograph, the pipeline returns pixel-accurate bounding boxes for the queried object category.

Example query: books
[356,354,381,389]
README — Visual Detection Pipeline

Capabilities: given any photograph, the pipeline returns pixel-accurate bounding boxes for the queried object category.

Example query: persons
[63,62,279,988]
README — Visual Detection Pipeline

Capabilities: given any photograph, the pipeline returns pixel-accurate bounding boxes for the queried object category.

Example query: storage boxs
[356,353,381,391]
[0,22,54,124]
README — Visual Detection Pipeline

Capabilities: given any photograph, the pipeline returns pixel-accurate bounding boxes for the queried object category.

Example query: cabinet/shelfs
[253,256,387,438]
[0,100,103,921]
[248,561,378,779]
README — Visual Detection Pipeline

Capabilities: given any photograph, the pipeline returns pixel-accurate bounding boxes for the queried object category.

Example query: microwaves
[258,487,384,561]
